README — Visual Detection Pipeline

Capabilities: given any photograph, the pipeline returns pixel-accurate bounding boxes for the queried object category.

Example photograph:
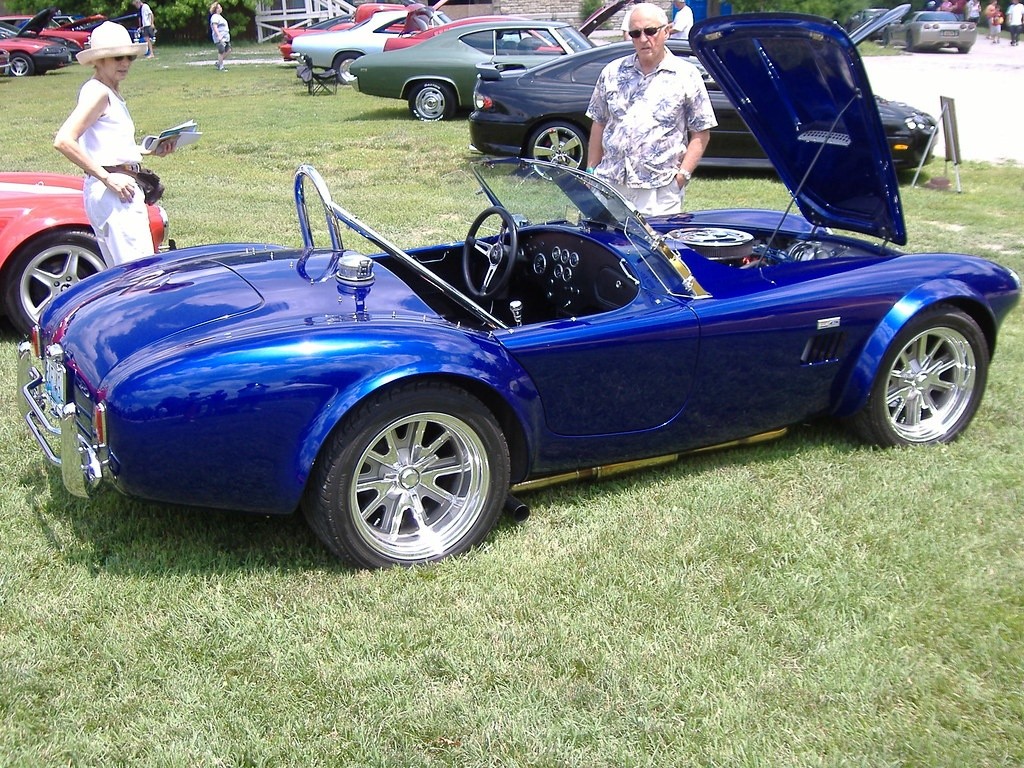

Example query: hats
[75,21,148,66]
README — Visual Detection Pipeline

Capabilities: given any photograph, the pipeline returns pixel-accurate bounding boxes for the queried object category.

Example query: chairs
[517,37,541,51]
[297,53,337,94]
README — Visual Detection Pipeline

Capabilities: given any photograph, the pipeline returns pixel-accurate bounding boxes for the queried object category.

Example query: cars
[0,7,157,77]
[347,21,599,121]
[382,15,565,54]
[278,0,448,62]
[845,9,902,40]
[882,10,978,54]
[308,14,354,30]
[469,3,939,182]
[290,12,453,85]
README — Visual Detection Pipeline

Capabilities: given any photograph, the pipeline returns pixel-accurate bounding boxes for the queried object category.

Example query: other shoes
[214,62,229,71]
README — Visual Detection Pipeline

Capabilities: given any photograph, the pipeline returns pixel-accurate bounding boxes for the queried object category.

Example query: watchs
[679,168,691,181]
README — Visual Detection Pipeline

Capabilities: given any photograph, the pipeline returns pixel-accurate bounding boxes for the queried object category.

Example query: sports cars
[16,11,1020,572]
[0,171,176,334]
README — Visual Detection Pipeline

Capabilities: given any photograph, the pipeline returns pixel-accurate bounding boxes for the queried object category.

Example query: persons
[985,0,1005,43]
[210,2,232,72]
[923,0,981,26]
[620,0,644,41]
[667,0,694,41]
[131,0,154,59]
[54,21,177,269]
[53,9,62,16]
[587,3,718,220]
[1006,0,1024,46]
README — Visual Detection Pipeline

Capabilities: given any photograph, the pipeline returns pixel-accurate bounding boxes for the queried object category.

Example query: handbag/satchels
[135,169,165,206]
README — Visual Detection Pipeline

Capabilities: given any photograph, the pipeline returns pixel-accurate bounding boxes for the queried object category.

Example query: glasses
[114,55,137,61]
[628,25,666,38]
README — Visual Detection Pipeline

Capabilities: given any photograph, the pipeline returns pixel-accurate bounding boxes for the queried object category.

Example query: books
[140,119,203,155]
